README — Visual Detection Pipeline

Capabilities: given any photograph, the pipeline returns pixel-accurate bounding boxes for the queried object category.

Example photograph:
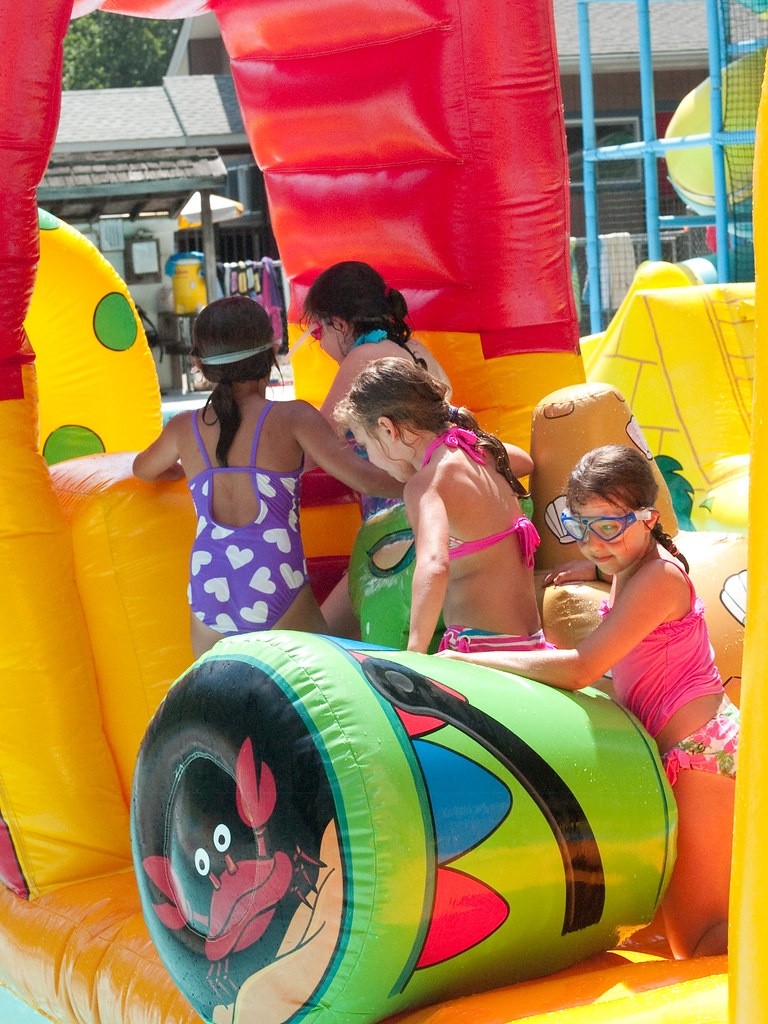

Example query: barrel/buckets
[171,258,208,314]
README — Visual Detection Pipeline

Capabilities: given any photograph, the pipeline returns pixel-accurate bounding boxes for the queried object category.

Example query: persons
[299,261,454,639]
[431,442,741,963]
[333,357,560,656]
[134,298,406,667]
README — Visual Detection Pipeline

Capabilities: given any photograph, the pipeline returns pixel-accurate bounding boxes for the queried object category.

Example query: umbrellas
[100,191,245,231]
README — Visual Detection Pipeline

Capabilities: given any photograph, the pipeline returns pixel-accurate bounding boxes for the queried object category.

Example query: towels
[581,231,636,312]
[223,256,284,355]
[569,236,583,323]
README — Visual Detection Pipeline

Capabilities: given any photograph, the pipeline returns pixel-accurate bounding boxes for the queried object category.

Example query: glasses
[308,319,324,340]
[561,508,637,541]
